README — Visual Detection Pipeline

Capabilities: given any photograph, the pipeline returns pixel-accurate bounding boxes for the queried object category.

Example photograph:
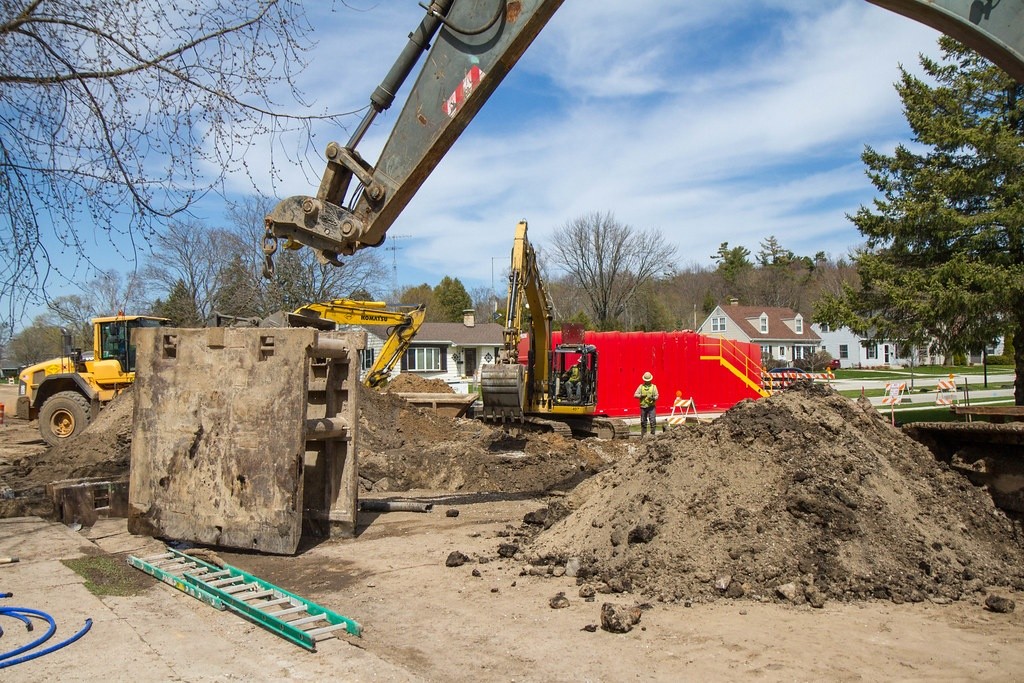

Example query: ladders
[125,545,365,652]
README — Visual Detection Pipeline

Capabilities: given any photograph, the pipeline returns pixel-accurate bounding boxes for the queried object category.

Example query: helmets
[642,372,653,381]
[577,356,582,362]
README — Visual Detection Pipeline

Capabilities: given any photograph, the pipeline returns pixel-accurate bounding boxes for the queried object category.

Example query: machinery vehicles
[230,0,1024,317]
[20,294,426,447]
[479,219,630,442]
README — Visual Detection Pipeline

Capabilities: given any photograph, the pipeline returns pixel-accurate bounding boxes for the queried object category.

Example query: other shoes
[567,394,574,401]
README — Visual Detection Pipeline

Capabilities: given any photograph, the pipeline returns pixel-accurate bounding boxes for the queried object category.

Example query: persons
[633,372,660,436]
[563,356,590,399]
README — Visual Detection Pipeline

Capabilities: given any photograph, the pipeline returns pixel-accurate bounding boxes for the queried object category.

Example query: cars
[821,352,841,370]
[764,367,806,391]
